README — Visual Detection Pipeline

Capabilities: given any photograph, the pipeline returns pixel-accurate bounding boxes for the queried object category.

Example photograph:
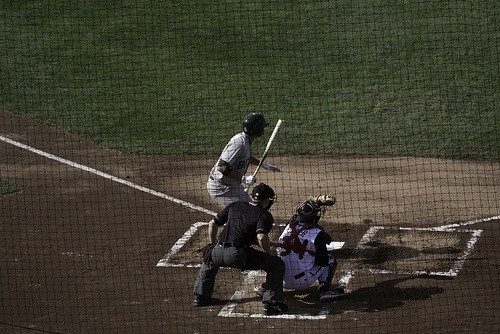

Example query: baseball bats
[243,118,286,193]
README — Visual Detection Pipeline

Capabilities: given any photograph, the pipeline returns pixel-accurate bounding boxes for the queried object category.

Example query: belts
[218,241,237,248]
[294,271,305,280]
[209,176,215,181]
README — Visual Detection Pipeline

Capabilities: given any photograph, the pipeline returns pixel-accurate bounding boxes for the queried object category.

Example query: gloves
[262,163,280,172]
[241,175,256,186]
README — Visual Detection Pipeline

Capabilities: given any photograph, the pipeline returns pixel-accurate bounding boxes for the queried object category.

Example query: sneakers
[263,301,288,315]
[193,293,212,307]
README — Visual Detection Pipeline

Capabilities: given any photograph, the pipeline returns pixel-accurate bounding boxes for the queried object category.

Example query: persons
[207,112,281,209]
[193,183,288,316]
[256,200,346,300]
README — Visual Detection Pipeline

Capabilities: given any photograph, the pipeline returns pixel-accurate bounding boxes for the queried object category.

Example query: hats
[252,183,274,199]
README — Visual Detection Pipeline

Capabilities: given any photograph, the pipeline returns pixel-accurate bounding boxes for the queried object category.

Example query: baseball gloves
[310,195,335,206]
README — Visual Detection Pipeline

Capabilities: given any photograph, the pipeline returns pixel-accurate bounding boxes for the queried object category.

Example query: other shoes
[254,283,265,298]
[320,290,345,303]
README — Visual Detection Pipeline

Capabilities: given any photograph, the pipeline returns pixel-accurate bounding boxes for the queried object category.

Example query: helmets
[296,201,321,224]
[242,113,269,135]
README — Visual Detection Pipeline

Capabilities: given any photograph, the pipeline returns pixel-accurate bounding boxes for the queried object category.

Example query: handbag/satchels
[202,241,217,265]
[236,247,261,272]
